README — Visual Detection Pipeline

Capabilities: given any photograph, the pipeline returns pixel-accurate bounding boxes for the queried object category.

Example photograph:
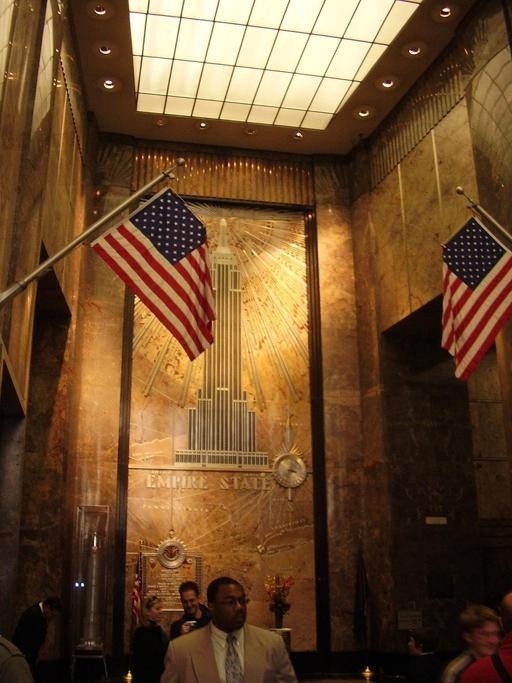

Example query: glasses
[214,600,247,607]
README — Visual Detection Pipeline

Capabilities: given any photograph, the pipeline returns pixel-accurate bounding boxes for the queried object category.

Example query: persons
[405,627,444,683]
[160,576,299,683]
[170,580,210,641]
[131,595,170,683]
[11,597,63,678]
[454,592,512,683]
[0,634,35,683]
[440,603,505,683]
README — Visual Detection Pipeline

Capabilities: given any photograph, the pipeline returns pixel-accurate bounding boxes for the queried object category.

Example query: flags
[91,188,218,363]
[440,216,512,386]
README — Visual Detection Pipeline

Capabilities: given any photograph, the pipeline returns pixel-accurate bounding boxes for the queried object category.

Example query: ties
[224,635,244,683]
